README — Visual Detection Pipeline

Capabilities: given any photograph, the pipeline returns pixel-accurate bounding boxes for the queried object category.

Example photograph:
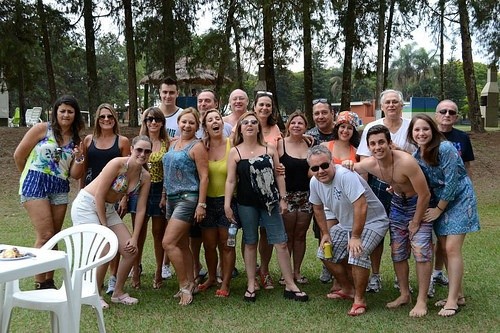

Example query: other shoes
[47,280,57,291]
[34,281,46,289]
[91,263,309,309]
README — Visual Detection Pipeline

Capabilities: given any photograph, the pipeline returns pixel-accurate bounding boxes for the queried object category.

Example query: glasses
[311,161,332,171]
[257,91,273,96]
[98,115,113,118]
[146,117,162,123]
[312,99,328,104]
[241,120,258,125]
[437,109,456,115]
[135,148,152,154]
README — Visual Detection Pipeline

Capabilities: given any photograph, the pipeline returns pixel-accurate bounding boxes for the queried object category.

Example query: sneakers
[433,272,449,287]
[366,274,382,293]
[427,280,435,297]
[394,274,413,292]
[319,263,332,283]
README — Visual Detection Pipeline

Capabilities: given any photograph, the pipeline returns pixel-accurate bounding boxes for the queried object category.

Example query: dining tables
[0,244,74,333]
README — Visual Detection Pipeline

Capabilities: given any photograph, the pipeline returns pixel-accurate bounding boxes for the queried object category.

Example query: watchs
[198,203,206,208]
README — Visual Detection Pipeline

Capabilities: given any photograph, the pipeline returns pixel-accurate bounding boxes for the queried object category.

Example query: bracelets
[437,206,443,212]
[281,195,288,200]
[74,155,85,164]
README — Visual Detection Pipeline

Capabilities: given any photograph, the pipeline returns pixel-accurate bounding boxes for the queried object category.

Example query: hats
[336,111,359,131]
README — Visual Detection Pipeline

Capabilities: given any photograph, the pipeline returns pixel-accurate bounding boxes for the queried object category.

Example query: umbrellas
[137,56,233,96]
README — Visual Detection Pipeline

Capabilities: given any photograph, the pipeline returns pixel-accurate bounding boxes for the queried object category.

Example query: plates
[0,251,31,261]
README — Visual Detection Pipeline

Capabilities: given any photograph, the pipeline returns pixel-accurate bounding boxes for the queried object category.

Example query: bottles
[227,223,237,246]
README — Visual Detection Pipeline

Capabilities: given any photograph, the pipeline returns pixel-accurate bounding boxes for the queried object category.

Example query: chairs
[8,106,43,128]
[2,224,118,333]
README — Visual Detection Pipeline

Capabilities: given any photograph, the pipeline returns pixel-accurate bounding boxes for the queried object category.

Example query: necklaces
[379,151,394,187]
[251,151,254,154]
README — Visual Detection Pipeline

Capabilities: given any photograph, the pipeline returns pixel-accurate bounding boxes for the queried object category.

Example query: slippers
[434,297,466,308]
[437,307,461,316]
[348,303,367,316]
[326,290,354,299]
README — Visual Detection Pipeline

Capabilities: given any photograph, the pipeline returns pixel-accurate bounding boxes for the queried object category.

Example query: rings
[203,216,204,218]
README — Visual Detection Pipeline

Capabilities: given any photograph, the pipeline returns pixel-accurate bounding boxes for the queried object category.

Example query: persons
[71,135,153,308]
[131,77,364,305]
[427,99,475,299]
[407,113,480,317]
[77,103,131,294]
[307,144,389,315]
[355,89,420,293]
[14,94,85,289]
[342,125,433,318]
